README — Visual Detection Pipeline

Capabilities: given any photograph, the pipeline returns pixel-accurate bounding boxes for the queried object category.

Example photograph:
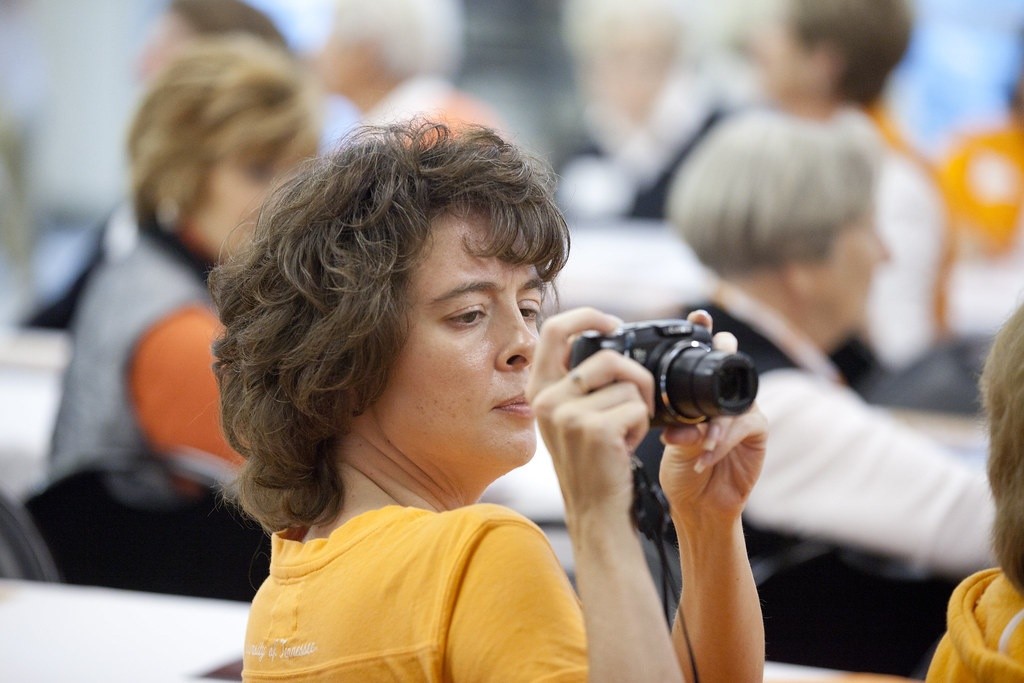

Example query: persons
[135,3,295,79]
[926,302,1024,681]
[20,28,319,605]
[597,103,995,625]
[305,1,1024,368]
[204,115,771,683]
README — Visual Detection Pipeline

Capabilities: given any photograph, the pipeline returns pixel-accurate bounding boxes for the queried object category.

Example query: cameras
[569,318,758,427]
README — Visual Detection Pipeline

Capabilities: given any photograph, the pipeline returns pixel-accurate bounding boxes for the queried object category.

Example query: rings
[571,370,585,393]
